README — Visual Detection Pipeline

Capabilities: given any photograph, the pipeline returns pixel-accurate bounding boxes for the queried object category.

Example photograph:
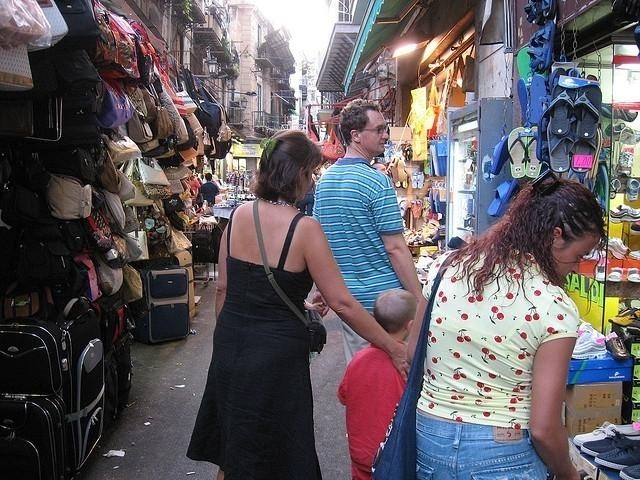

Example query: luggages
[37,294,108,477]
[130,262,192,346]
[0,318,77,480]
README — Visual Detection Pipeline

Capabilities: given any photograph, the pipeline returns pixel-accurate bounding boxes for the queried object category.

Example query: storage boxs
[564,352,633,440]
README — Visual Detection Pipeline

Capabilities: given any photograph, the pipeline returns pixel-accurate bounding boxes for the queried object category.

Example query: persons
[311,98,421,368]
[412,175,609,480]
[336,288,423,478]
[293,170,318,218]
[198,172,220,207]
[186,127,413,479]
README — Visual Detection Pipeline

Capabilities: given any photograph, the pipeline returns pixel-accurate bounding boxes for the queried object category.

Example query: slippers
[486,67,609,218]
[390,142,446,232]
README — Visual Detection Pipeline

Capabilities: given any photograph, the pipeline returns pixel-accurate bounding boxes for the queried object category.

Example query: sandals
[526,19,556,75]
[524,0,558,26]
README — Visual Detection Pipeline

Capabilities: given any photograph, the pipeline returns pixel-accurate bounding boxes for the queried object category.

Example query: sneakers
[607,305,640,328]
[580,432,640,456]
[594,445,640,471]
[572,419,640,449]
[618,464,640,479]
[582,103,640,284]
[606,334,630,360]
[571,323,608,360]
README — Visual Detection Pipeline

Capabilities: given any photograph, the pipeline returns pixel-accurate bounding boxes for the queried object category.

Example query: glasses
[358,125,390,135]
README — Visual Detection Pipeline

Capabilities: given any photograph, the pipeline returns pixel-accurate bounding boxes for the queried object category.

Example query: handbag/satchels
[461,54,477,93]
[0,0,234,345]
[304,308,328,354]
[421,105,449,139]
[368,363,426,480]
